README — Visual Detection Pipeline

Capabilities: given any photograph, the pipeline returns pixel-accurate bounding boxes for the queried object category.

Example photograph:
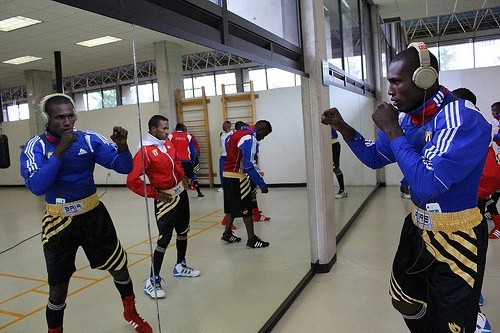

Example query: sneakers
[488,227,500,239]
[478,294,484,306]
[221,231,241,242]
[401,192,411,198]
[334,191,347,199]
[123,312,152,333]
[217,188,223,192]
[172,259,200,277]
[475,312,491,333]
[253,216,270,221]
[198,193,204,197]
[222,219,236,230]
[190,186,196,190]
[485,212,491,219]
[246,236,269,248]
[142,275,165,299]
[48,327,63,333]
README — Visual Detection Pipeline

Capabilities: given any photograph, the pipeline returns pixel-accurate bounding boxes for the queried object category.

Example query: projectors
[39,93,78,129]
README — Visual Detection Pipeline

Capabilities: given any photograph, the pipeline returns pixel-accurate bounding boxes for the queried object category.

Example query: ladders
[176,86,217,189]
[221,80,264,176]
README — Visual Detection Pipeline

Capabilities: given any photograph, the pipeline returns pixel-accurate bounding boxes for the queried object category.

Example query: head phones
[406,42,438,89]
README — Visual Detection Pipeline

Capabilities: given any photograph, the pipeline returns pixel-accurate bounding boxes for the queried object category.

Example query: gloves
[262,188,268,193]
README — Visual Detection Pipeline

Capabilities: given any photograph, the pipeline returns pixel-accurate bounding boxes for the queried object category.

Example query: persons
[168,123,204,197]
[20,93,154,333]
[127,115,200,299]
[331,128,348,199]
[320,47,493,333]
[217,121,271,229]
[221,120,272,248]
[400,88,500,306]
